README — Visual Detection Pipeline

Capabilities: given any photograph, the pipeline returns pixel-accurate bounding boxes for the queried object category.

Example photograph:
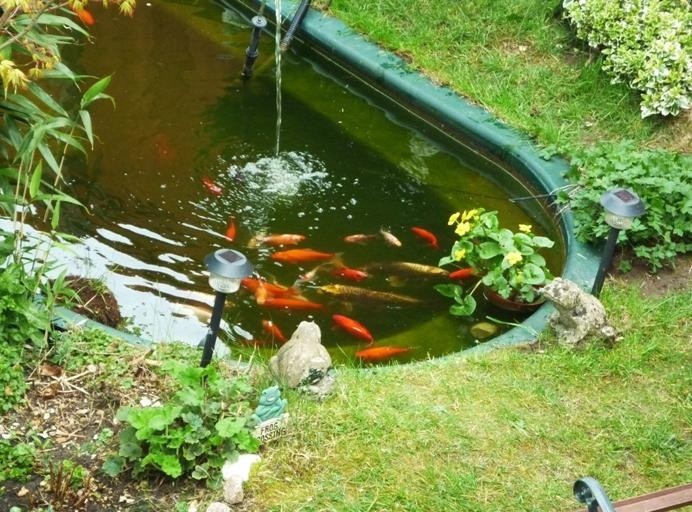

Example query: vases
[481,285,543,314]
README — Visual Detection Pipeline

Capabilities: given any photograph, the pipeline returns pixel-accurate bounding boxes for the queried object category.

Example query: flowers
[434,208,554,316]
